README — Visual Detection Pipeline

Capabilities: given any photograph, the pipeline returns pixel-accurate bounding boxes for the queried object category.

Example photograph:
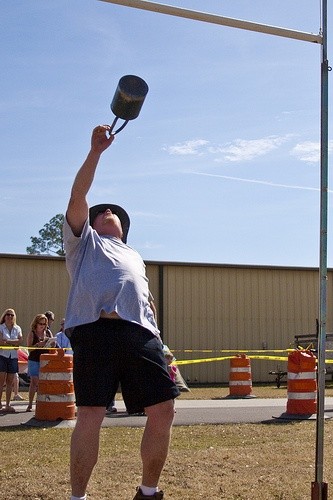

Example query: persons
[25,314,58,412]
[62,124,192,500]
[56,318,73,354]
[0,309,23,416]
[44,311,55,329]
[106,394,117,415]
[13,360,25,401]
